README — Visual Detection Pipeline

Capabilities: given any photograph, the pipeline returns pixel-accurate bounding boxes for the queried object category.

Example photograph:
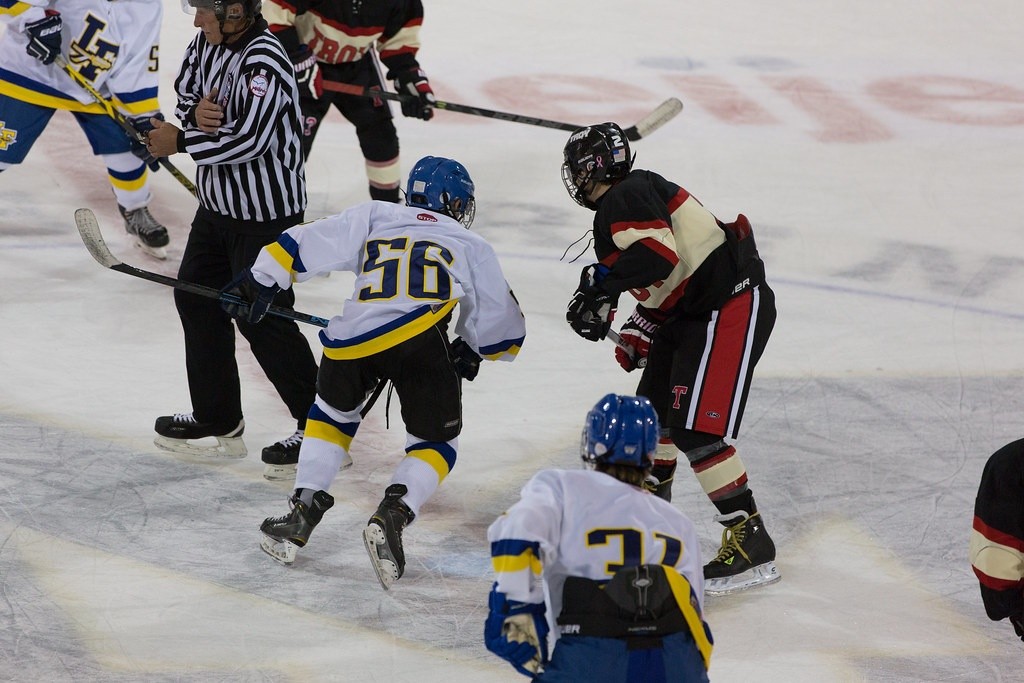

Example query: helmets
[563,120,635,208]
[405,155,475,229]
[580,393,658,473]
[188,0,262,22]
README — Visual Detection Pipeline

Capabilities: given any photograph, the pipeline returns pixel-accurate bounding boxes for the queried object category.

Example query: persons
[217,157,526,580]
[560,122,776,579]
[0,1,169,247]
[261,0,437,205]
[146,1,319,465]
[971,438,1024,643]
[487,393,714,683]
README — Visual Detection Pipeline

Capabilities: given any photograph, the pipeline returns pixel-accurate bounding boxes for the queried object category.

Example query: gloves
[218,265,282,322]
[384,52,433,120]
[127,113,170,171]
[449,334,481,382]
[484,582,551,677]
[288,44,323,100]
[564,263,623,343]
[618,303,662,371]
[24,9,62,64]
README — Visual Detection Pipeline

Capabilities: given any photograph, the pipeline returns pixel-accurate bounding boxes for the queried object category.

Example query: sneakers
[364,483,415,592]
[154,410,247,458]
[262,427,355,481]
[703,509,781,597]
[118,193,170,260]
[259,491,335,563]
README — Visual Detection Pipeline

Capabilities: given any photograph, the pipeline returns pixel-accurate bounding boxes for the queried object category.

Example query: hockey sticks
[581,309,640,361]
[321,81,686,143]
[72,206,330,327]
[50,58,197,196]
[504,621,551,670]
